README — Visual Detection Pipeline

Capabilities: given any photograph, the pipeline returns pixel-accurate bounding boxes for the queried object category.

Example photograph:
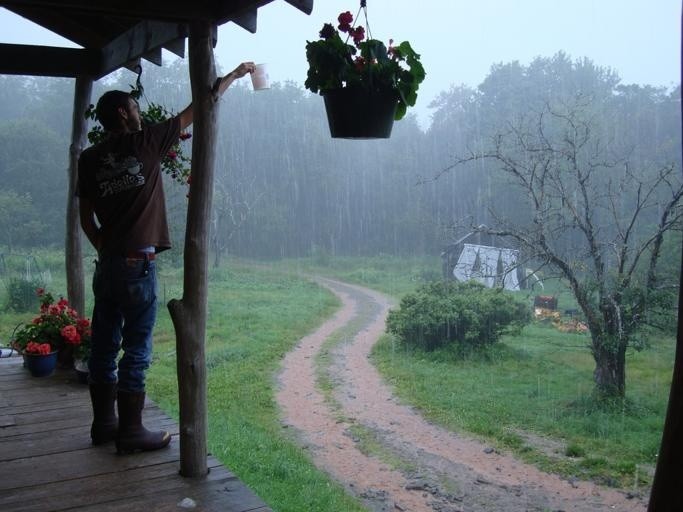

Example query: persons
[74,61,257,455]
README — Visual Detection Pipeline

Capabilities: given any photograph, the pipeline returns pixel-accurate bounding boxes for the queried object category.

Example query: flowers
[5,287,92,379]
[300,9,425,121]
[82,85,194,186]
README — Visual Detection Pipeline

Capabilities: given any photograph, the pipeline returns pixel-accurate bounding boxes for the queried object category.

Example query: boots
[88,380,119,445]
[115,390,171,457]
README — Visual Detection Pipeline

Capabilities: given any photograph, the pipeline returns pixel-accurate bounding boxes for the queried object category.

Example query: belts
[97,249,158,263]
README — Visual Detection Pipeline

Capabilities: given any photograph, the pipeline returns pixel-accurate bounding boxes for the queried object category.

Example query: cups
[250,63,270,92]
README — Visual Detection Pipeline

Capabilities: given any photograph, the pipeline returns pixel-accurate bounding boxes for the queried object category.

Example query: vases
[317,89,398,139]
